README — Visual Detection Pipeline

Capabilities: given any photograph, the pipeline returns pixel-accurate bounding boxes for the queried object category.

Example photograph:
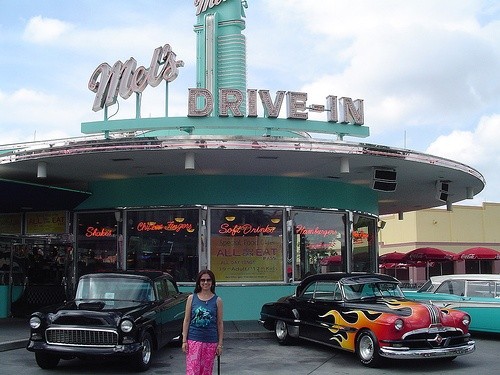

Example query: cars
[258,271,476,368]
[394,274,500,338]
[26,268,193,373]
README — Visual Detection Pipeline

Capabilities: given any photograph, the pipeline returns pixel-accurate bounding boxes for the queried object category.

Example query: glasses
[200,278,211,282]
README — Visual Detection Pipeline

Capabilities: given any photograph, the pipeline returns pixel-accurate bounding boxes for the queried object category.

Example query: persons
[181,269,223,375]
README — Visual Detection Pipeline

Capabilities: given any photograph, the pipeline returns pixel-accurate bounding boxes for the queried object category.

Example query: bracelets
[182,341,187,343]
[217,344,224,349]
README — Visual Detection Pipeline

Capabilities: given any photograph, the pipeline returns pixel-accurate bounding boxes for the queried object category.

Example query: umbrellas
[428,250,458,276]
[458,247,500,274]
[378,251,426,278]
[402,247,448,279]
